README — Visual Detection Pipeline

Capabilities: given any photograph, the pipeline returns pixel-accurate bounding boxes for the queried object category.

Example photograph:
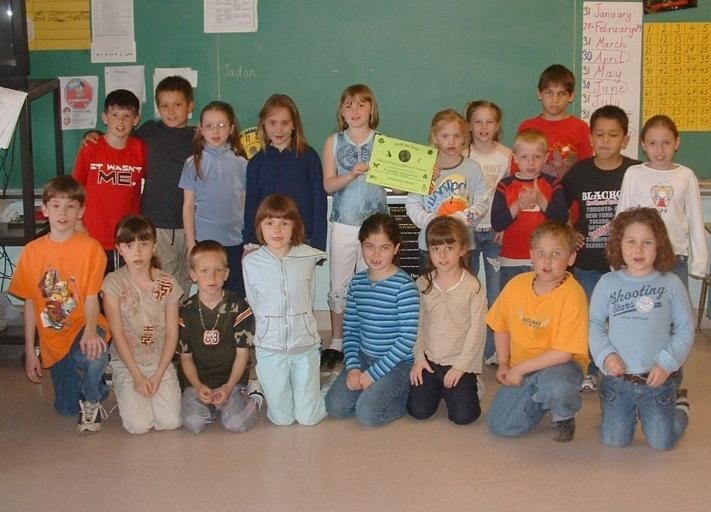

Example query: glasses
[203,121,229,130]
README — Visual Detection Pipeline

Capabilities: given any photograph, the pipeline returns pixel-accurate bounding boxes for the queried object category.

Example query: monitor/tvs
[0,0,30,92]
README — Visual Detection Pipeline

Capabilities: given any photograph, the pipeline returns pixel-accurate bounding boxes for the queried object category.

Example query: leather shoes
[320,348,343,368]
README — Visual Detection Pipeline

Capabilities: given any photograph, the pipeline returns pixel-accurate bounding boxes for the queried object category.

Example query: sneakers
[484,351,497,365]
[102,364,113,386]
[248,377,263,396]
[676,388,690,405]
[552,417,576,441]
[76,392,109,433]
[476,376,484,400]
[581,375,599,391]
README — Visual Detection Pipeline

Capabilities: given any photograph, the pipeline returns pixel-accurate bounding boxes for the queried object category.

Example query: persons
[406,216,489,426]
[480,224,588,445]
[70,87,149,390]
[403,109,492,282]
[76,72,201,292]
[100,212,186,436]
[614,113,707,397]
[561,104,645,393]
[491,128,564,296]
[236,196,329,427]
[322,215,422,431]
[588,204,694,448]
[462,98,515,367]
[7,175,110,435]
[176,99,250,302]
[509,60,596,186]
[242,92,328,258]
[321,84,437,365]
[176,238,266,434]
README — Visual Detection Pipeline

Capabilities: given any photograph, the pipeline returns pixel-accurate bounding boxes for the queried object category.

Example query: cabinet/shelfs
[0,79,66,371]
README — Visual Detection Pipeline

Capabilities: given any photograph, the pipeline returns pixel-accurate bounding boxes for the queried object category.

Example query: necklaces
[533,272,569,290]
[198,288,225,335]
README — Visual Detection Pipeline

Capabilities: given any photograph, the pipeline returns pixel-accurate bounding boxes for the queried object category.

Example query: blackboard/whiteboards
[637,0,711,185]
[0,0,583,202]
[581,0,644,162]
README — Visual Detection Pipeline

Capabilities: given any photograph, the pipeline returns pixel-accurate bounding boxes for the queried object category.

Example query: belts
[619,372,678,385]
